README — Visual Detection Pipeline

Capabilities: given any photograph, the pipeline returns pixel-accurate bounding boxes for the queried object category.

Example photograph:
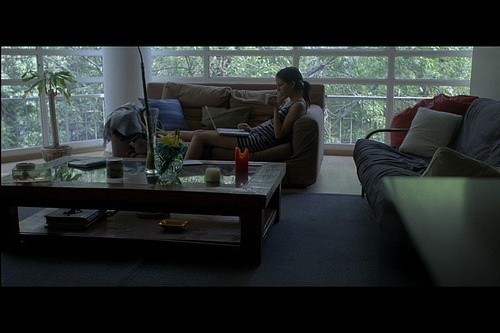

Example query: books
[216,128,250,137]
[43,208,107,227]
[68,157,106,168]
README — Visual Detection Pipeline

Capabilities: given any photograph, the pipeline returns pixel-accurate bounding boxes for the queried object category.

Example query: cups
[106,158,124,184]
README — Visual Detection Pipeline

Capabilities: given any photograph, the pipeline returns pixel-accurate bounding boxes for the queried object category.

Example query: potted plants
[134,47,160,174]
[21,69,73,161]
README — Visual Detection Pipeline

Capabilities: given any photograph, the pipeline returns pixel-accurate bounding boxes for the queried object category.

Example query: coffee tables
[0,156,286,266]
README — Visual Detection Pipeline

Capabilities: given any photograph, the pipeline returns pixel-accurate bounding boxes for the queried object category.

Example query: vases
[154,154,183,178]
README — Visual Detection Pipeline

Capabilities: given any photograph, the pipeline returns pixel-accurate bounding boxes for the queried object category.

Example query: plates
[157,219,189,228]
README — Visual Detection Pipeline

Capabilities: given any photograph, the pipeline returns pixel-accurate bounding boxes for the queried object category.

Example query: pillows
[420,146,500,179]
[200,105,252,128]
[137,99,184,130]
[399,107,461,160]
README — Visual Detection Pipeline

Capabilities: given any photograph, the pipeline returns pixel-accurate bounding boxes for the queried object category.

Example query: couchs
[353,94,500,286]
[108,82,325,186]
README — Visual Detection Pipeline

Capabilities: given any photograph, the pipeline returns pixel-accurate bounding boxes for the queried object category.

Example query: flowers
[159,130,188,154]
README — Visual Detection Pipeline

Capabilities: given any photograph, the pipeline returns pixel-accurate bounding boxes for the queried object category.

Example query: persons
[155,67,311,160]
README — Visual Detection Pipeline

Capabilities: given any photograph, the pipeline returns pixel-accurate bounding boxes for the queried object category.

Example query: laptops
[205,106,249,136]
